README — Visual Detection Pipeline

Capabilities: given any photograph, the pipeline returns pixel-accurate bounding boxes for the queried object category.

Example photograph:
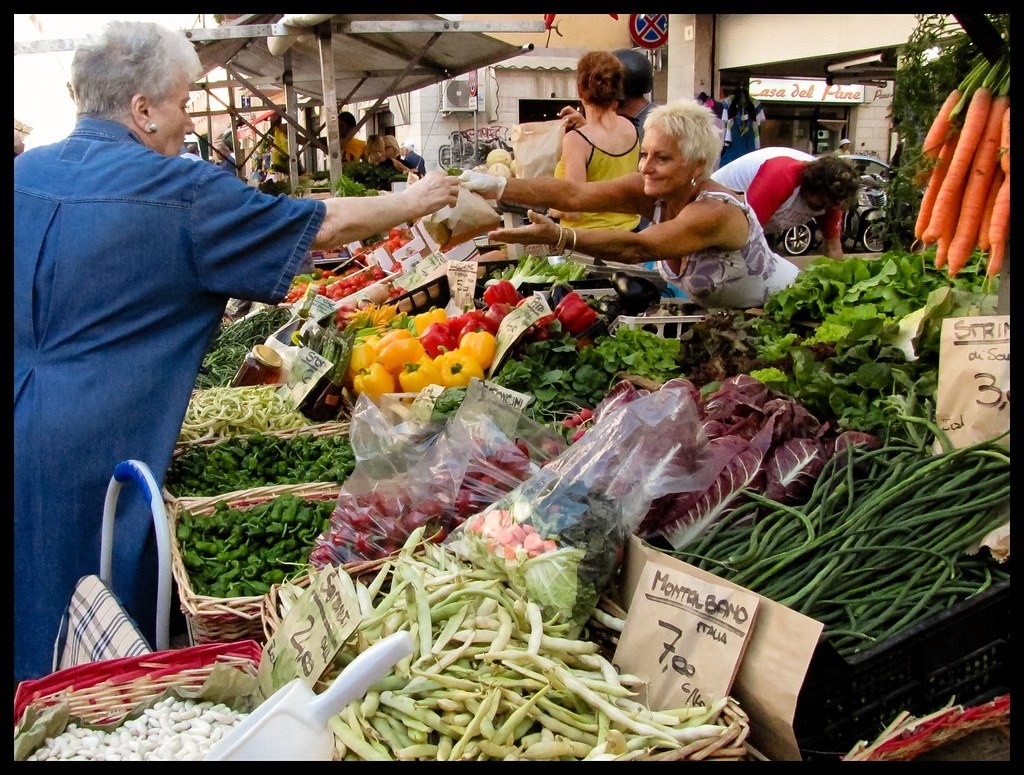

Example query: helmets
[612,48,654,96]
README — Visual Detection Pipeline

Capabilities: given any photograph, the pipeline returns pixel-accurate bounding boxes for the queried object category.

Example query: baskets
[868,191,885,208]
[13,252,1011,762]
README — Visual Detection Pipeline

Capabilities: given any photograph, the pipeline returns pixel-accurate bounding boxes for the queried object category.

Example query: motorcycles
[780,177,888,255]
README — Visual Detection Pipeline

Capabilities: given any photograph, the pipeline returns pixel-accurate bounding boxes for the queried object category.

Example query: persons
[709,145,860,261]
[179,109,428,195]
[552,49,673,235]
[832,137,852,155]
[455,97,802,314]
[12,12,477,708]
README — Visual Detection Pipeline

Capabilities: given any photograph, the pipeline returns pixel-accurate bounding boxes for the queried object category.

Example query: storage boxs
[9,214,1015,766]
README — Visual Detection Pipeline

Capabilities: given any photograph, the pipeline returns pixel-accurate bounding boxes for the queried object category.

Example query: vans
[181,133,203,160]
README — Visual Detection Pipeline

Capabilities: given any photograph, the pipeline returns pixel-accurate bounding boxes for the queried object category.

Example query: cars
[833,154,926,204]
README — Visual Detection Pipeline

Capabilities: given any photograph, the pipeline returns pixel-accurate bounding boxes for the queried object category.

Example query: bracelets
[543,221,577,259]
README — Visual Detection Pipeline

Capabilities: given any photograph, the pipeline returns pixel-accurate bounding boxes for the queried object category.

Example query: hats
[839,138,851,148]
